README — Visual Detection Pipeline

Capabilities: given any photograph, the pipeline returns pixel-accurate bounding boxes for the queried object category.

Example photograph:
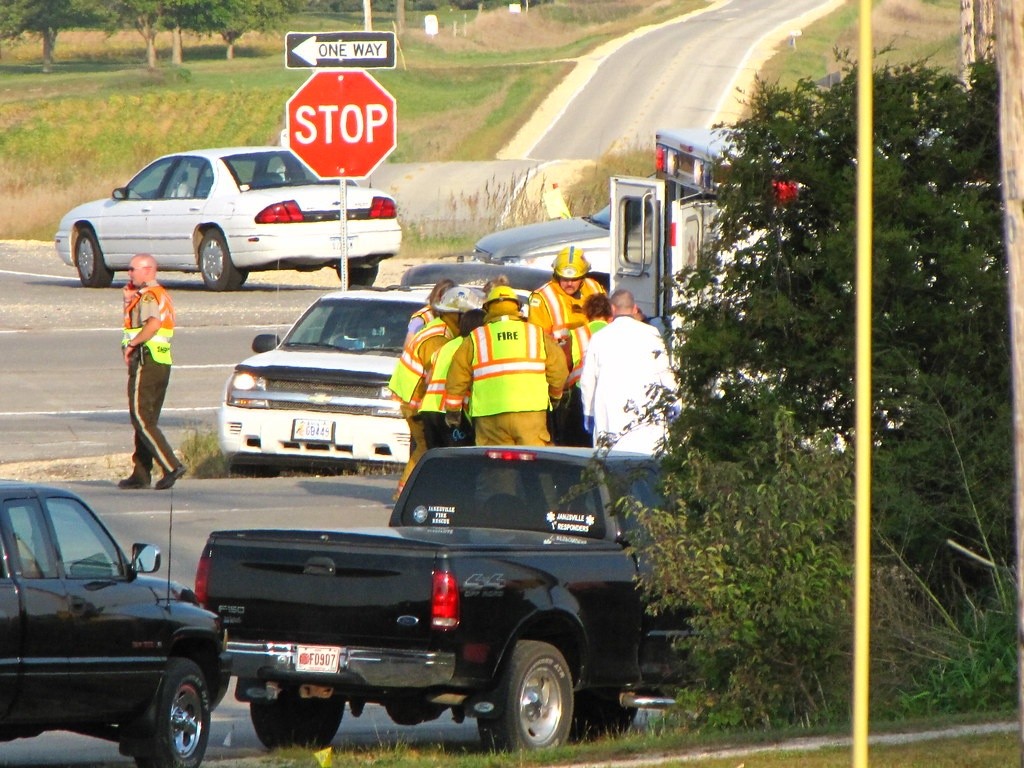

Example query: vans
[609,123,842,415]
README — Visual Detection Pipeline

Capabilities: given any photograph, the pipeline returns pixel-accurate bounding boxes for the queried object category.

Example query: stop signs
[285,68,398,182]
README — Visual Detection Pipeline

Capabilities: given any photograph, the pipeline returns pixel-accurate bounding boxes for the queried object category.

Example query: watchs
[127,340,135,348]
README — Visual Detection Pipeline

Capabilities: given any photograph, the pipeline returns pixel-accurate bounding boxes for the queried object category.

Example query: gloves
[445,410,461,428]
[550,396,561,409]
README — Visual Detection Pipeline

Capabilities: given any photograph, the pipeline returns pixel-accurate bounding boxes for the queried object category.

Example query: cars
[56,147,404,294]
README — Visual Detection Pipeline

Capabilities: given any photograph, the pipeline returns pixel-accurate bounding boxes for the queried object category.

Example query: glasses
[126,265,151,272]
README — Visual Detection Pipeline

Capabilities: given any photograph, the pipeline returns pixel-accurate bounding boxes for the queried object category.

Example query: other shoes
[156,463,186,490]
[392,491,400,501]
[118,472,151,489]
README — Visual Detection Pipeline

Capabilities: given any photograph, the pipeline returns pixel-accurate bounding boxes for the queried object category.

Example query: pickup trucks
[188,445,701,753]
[0,483,235,767]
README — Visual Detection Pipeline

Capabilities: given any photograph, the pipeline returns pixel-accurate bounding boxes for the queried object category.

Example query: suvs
[473,167,661,277]
[221,260,609,476]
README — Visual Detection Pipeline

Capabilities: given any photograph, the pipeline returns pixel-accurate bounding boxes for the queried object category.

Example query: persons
[115,253,187,491]
[388,245,683,505]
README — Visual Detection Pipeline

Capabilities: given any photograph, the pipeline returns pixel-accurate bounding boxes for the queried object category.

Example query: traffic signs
[284,30,396,72]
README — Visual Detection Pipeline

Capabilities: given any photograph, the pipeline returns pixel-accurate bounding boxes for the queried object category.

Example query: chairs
[16,535,46,580]
[479,496,546,532]
[564,492,598,533]
[355,319,396,346]
[262,173,284,184]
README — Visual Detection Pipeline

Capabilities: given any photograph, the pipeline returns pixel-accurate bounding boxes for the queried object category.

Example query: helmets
[551,246,591,278]
[481,284,523,312]
[435,287,483,313]
